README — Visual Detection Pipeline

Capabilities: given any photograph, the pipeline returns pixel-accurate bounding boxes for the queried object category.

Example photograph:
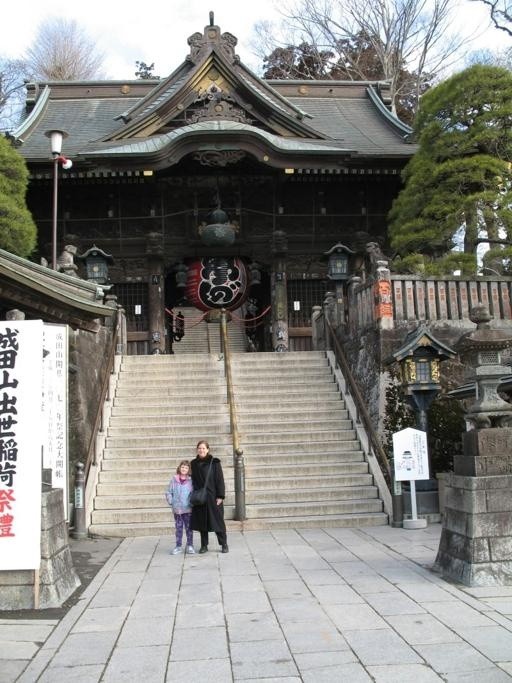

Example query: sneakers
[185,545,195,555]
[170,546,183,555]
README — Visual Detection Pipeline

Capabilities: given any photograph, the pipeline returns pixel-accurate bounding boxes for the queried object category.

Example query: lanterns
[186,259,252,323]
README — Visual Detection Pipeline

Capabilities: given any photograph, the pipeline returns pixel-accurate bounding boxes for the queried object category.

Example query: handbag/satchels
[190,488,207,507]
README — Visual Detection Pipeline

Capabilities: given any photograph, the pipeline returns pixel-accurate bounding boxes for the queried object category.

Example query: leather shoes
[221,544,229,554]
[199,545,208,554]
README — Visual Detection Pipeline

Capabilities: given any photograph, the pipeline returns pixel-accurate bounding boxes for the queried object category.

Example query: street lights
[44,129,70,271]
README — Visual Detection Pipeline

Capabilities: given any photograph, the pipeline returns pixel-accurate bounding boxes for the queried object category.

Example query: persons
[190,441,228,553]
[165,461,194,555]
